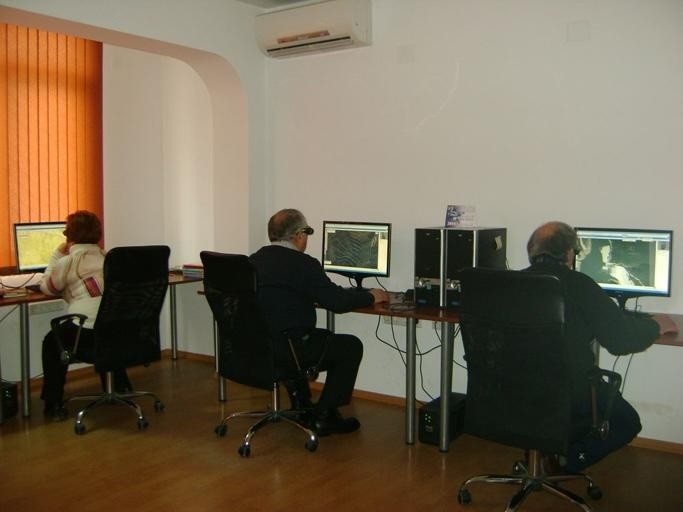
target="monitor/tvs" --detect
[322,221,391,291]
[573,227,673,317]
[14,222,67,272]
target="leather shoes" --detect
[42,398,70,424]
[287,397,361,437]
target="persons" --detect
[520,222,677,473]
[248,209,390,436]
[40,211,132,421]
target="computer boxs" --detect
[413,227,486,308]
[445,228,507,306]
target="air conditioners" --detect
[256,0,373,58]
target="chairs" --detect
[197,251,335,458]
[456,267,622,512]
[51,246,171,435]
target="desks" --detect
[0,266,220,418]
[417,289,683,453]
[195,286,417,445]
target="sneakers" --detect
[543,455,562,486]
